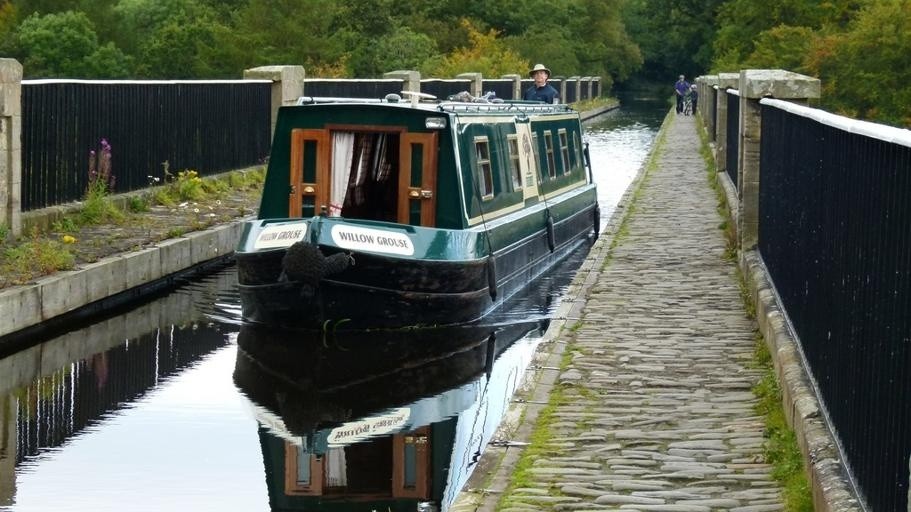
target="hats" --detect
[529,64,552,80]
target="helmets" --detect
[691,84,698,89]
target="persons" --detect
[689,85,698,114]
[521,63,561,104]
[674,74,692,114]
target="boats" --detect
[232,80,603,325]
[227,315,552,511]
